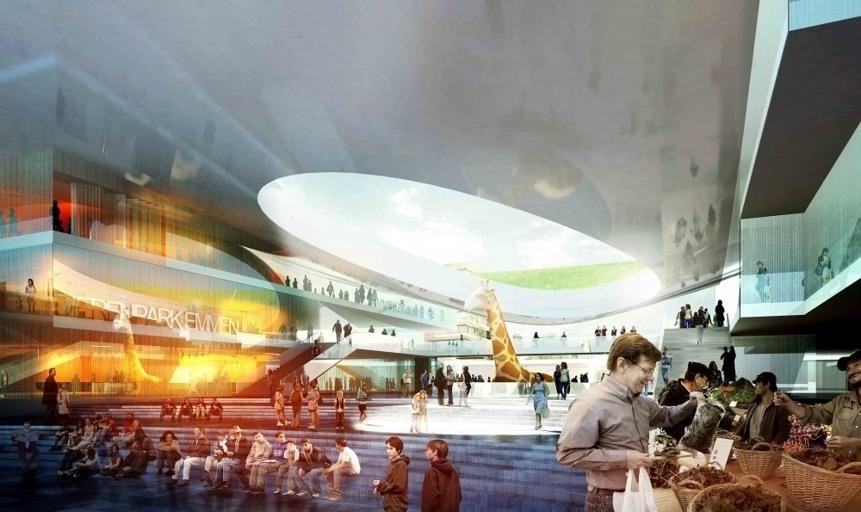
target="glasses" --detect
[629,359,656,376]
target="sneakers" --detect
[166,479,189,486]
[203,479,265,495]
[273,490,343,501]
[277,421,291,426]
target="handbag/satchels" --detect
[566,385,570,393]
[543,407,550,418]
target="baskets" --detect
[668,429,861,512]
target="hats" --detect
[688,363,713,383]
[753,372,776,384]
[837,351,861,371]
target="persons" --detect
[1,199,858,510]
